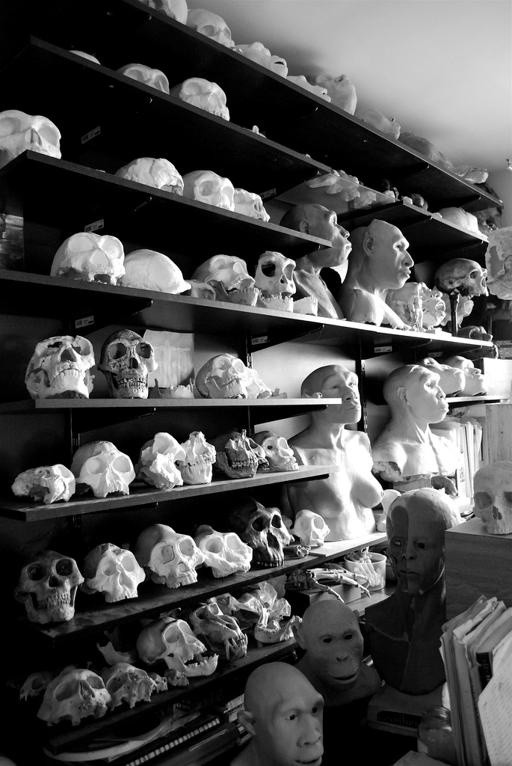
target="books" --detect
[437,594,512,765]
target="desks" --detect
[446,506,511,620]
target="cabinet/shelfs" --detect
[1,0,510,766]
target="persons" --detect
[444,356,487,397]
[364,486,468,695]
[291,599,383,709]
[276,200,352,321]
[334,217,414,330]
[285,365,385,545]
[226,662,326,766]
[371,361,468,481]
